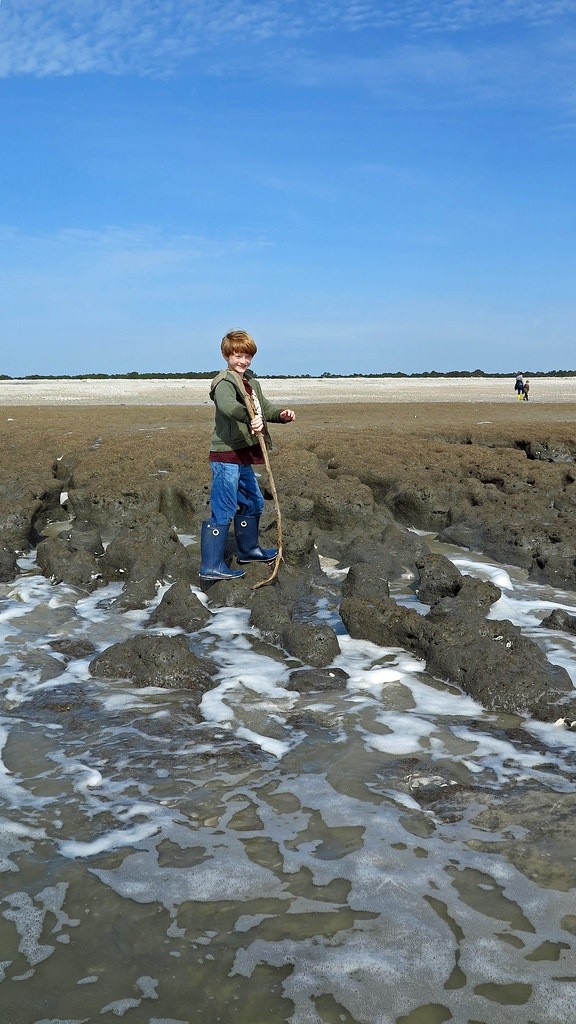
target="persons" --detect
[202,328,296,582]
[515,372,524,402]
[523,380,530,400]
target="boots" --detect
[235,511,279,563]
[199,520,246,582]
